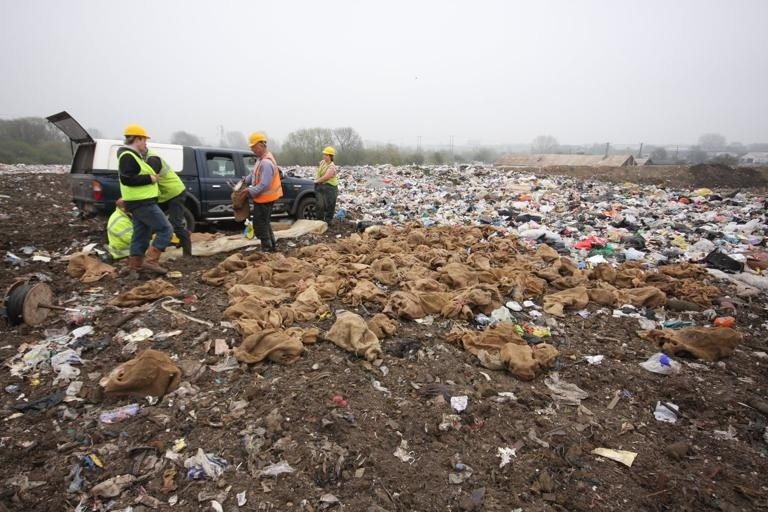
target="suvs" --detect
[42,110,322,231]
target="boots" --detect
[181,240,193,262]
[256,233,276,253]
[141,245,168,274]
[129,256,150,273]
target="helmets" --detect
[322,147,336,156]
[248,132,267,147]
[123,124,151,139]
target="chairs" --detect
[206,158,225,178]
[225,159,235,178]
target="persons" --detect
[115,124,175,274]
[313,146,339,225]
[238,133,284,253]
[106,197,133,252]
[139,146,192,262]
